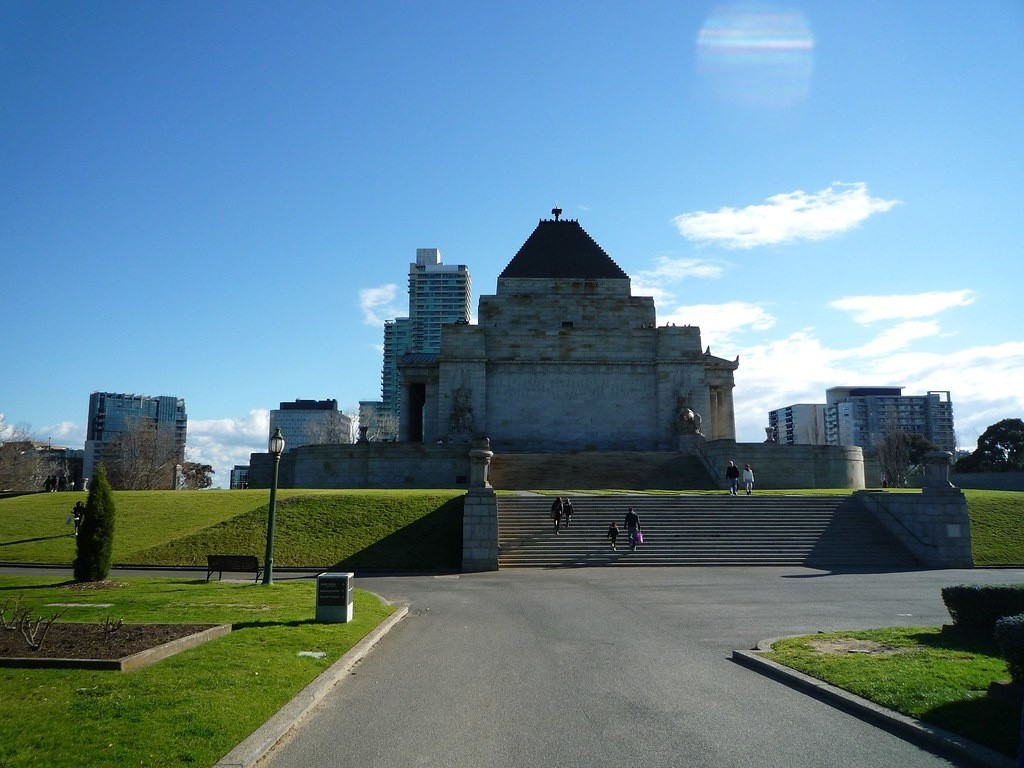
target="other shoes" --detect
[733,491,736,495]
[747,491,751,495]
[566,524,569,527]
[556,532,559,535]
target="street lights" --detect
[260,423,286,586]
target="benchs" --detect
[204,554,263,583]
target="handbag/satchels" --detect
[637,531,643,543]
[551,512,554,519]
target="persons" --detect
[455,319,468,324]
[43,474,75,492]
[551,497,563,534]
[726,461,740,495]
[608,521,618,551]
[70,501,85,535]
[624,508,641,551]
[564,498,573,527]
[743,465,754,495]
